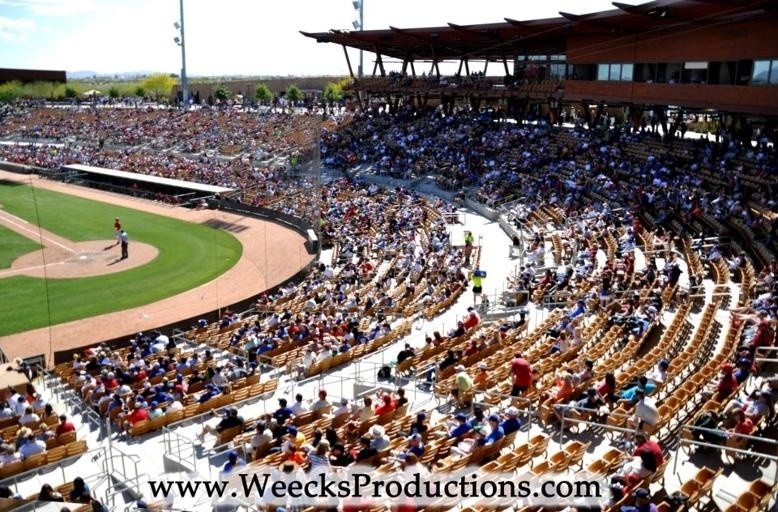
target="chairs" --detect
[0,238,352,511]
[352,111,778,512]
[0,102,352,226]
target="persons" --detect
[0,291,778,508]
[264,217,778,294]
[120,230,129,260]
[0,66,778,218]
[113,217,122,245]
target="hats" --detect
[228,451,238,459]
[235,358,256,367]
[408,433,422,440]
[658,359,668,367]
[454,413,466,420]
[205,384,213,388]
[369,424,385,438]
[487,406,518,421]
[454,362,487,371]
[137,363,182,406]
[16,425,32,438]
[417,412,425,419]
[721,350,751,370]
[287,427,297,433]
